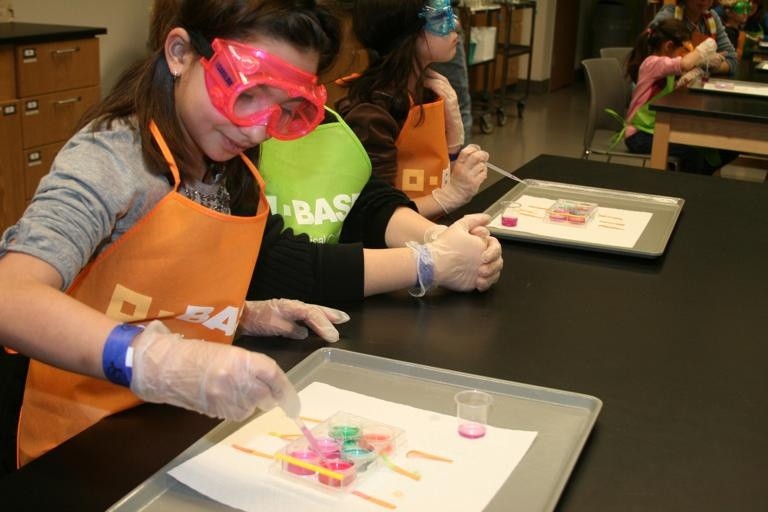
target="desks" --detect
[12,151,768,511]
[647,82,768,174]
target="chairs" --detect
[579,46,681,174]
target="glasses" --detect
[732,3,752,15]
[421,0,458,36]
[677,41,693,56]
[193,38,330,140]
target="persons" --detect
[433,0,473,143]
[0,0,350,512]
[623,0,737,110]
[621,16,740,175]
[334,0,492,216]
[711,0,768,64]
[240,2,507,317]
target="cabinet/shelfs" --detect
[456,0,537,117]
[0,38,102,237]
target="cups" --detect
[500,201,523,227]
[702,72,710,82]
[454,390,494,438]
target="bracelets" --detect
[415,243,434,291]
[101,318,147,384]
